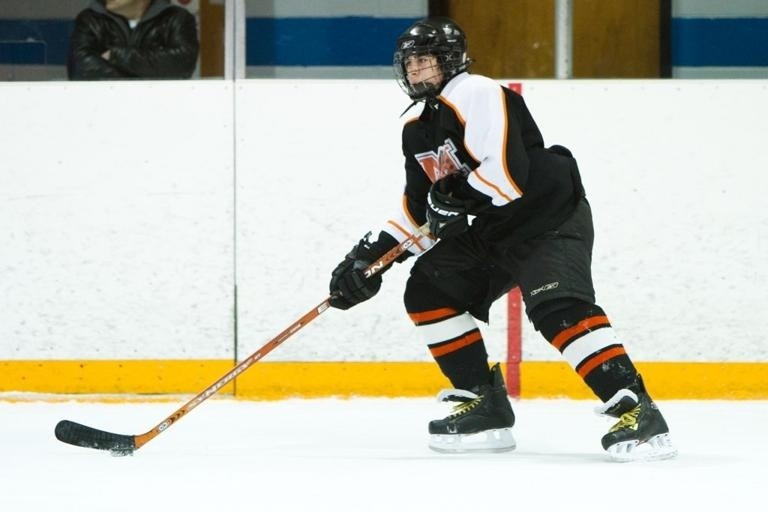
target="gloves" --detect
[330,231,393,309]
[426,172,491,239]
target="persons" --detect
[329,16,677,463]
[67,2,199,81]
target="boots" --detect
[594,373,668,450]
[429,363,514,433]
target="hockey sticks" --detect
[56,224,433,457]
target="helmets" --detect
[394,17,476,99]
